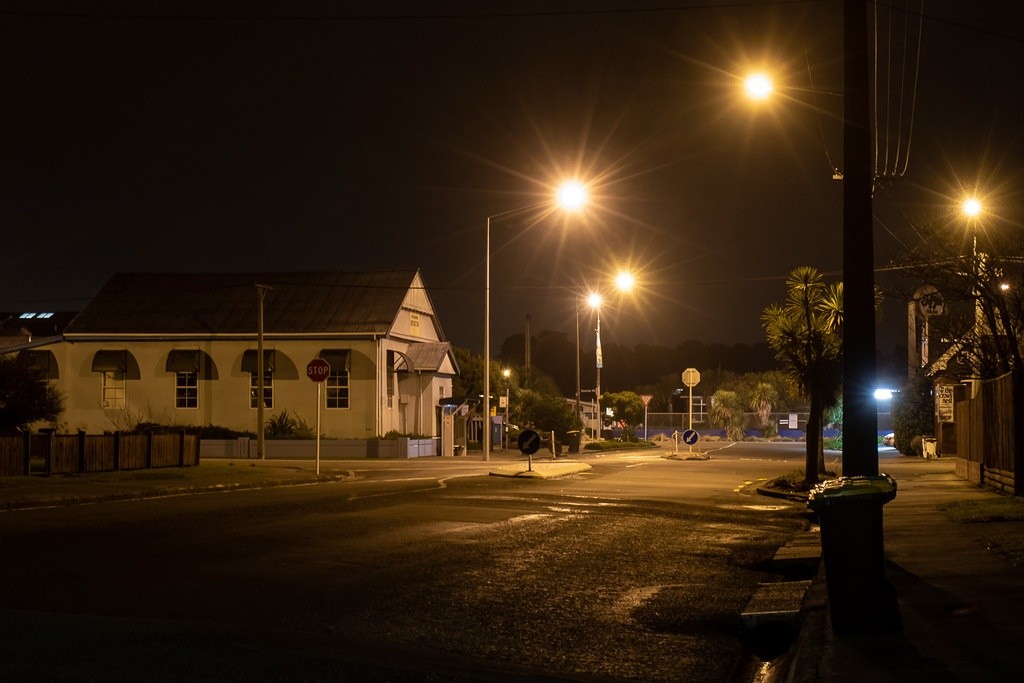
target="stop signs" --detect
[307,358,331,382]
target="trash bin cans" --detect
[805,471,896,637]
[567,431,582,452]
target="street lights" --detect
[503,369,512,450]
[482,184,589,462]
[588,293,603,439]
